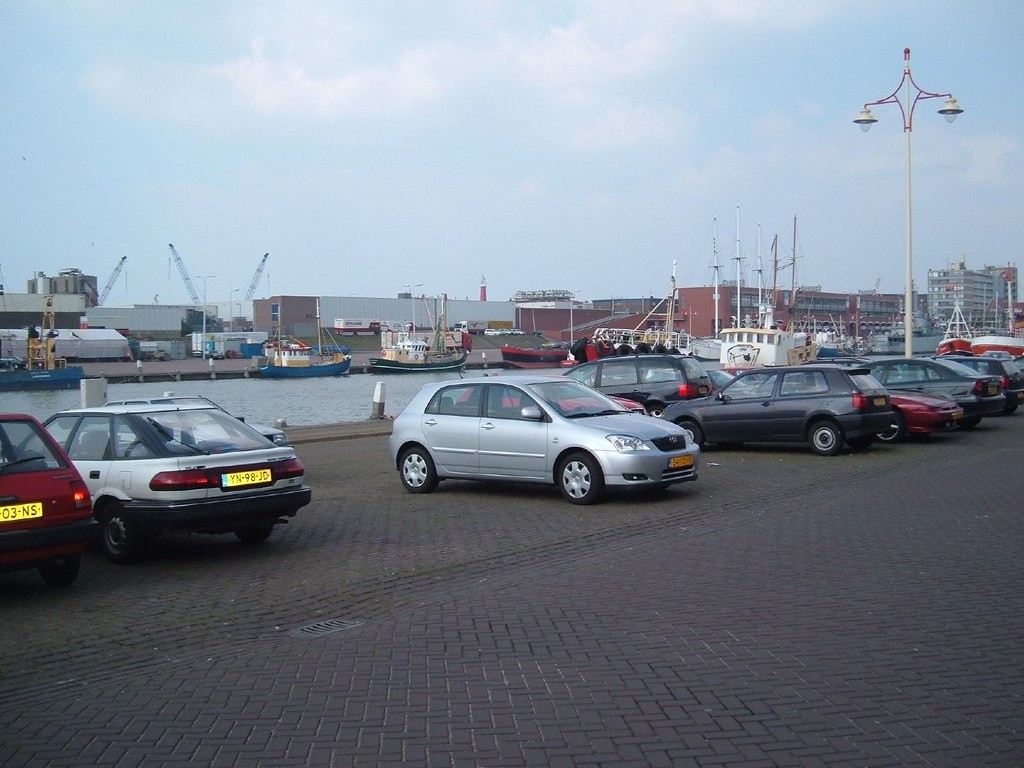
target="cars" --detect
[390,375,701,504]
[704,369,754,394]
[854,358,1024,430]
[876,392,970,444]
[0,393,313,588]
[888,328,905,343]
[457,376,649,420]
[208,350,225,360]
[721,366,768,382]
[0,357,28,369]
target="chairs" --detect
[80,431,109,455]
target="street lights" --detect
[402,283,423,334]
[569,289,582,348]
[852,46,964,355]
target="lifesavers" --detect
[668,348,681,354]
[652,344,668,353]
[595,341,615,357]
[570,337,587,355]
[414,354,418,360]
[614,344,635,356]
[381,351,386,357]
[635,342,652,354]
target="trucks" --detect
[454,321,488,336]
[334,318,416,337]
[380,331,472,353]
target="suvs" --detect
[660,364,897,457]
[562,353,716,419]
[752,357,902,395]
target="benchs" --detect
[430,388,480,415]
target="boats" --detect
[936,302,973,358]
[969,270,1024,358]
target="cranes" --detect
[99,242,279,311]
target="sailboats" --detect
[257,298,355,381]
[369,295,470,375]
[484,206,876,368]
